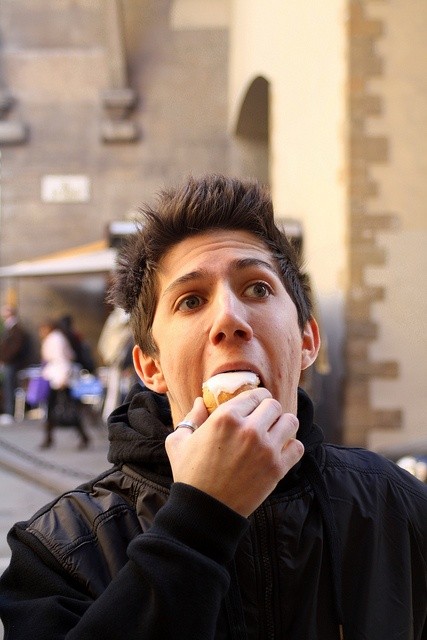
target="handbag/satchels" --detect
[26,377,50,405]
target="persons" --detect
[0,176,427,640]
[39,314,93,450]
[0,304,26,424]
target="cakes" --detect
[199,370,261,415]
